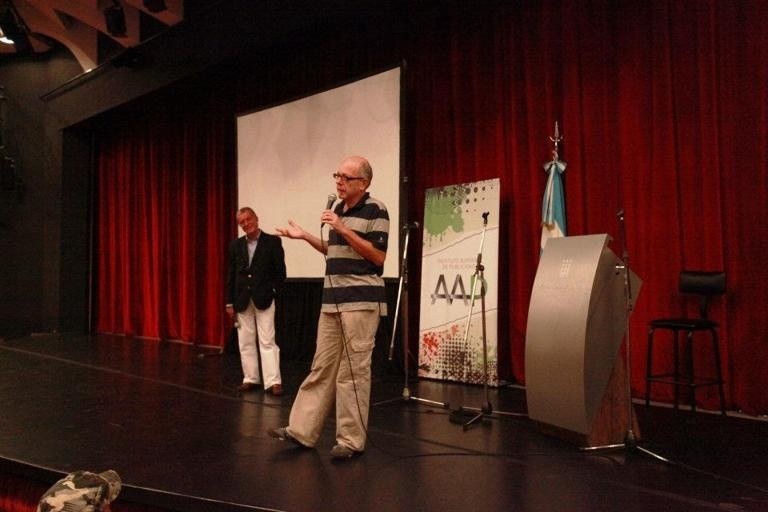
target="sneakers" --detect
[330,444,353,459]
[268,426,307,449]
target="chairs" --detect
[644,271,728,418]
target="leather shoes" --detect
[238,384,251,390]
[272,384,282,395]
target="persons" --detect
[223,206,285,395]
[265,156,390,459]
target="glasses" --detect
[334,172,364,182]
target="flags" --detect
[538,163,568,257]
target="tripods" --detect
[460,211,529,429]
[576,210,672,466]
[370,229,451,408]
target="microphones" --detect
[320,194,337,227]
[231,317,240,328]
[401,221,420,228]
